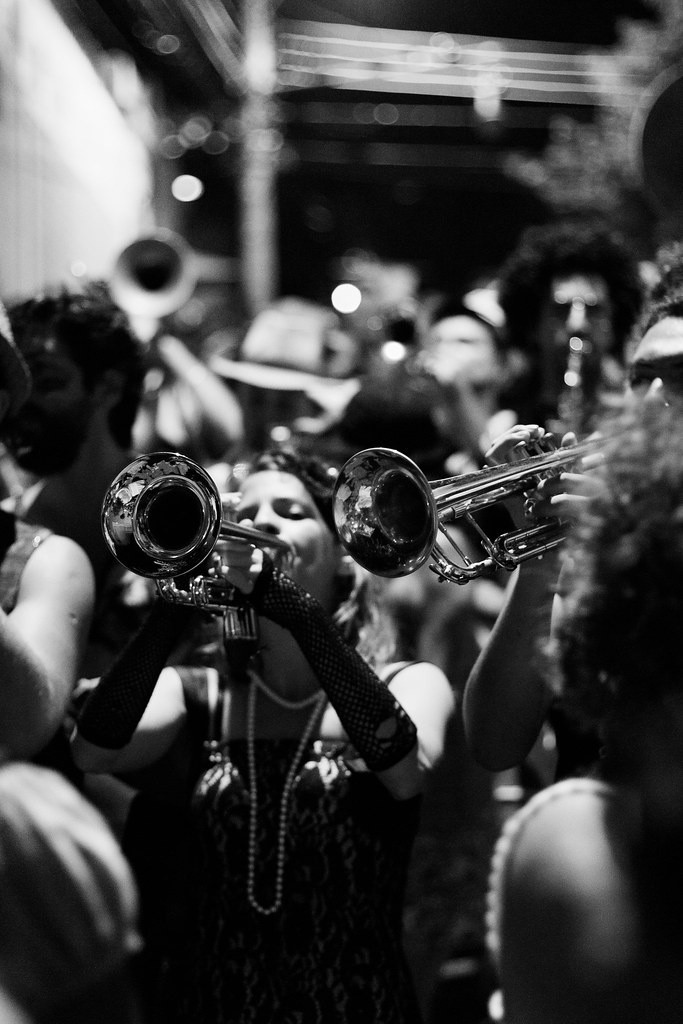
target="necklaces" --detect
[246,670,326,917]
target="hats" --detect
[0,297,31,404]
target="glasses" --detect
[628,351,683,396]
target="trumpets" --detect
[99,452,298,611]
[332,424,648,585]
[112,225,202,314]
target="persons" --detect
[0,56,683,1024]
